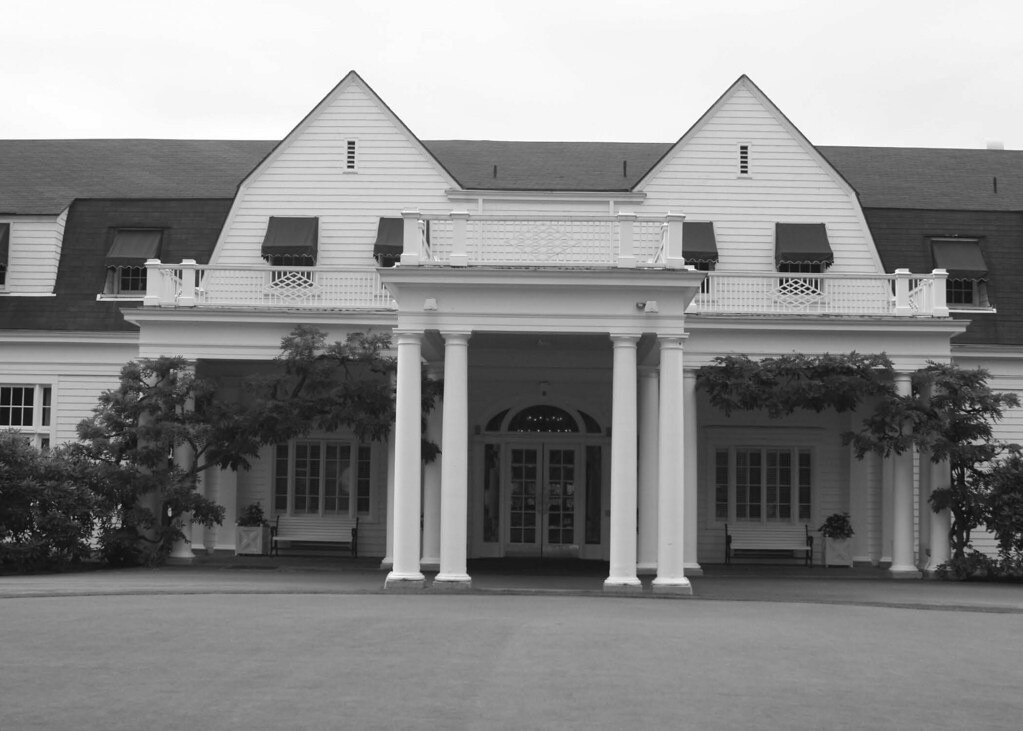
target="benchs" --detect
[721,521,815,569]
[270,515,361,558]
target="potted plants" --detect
[234,503,272,556]
[817,515,854,569]
